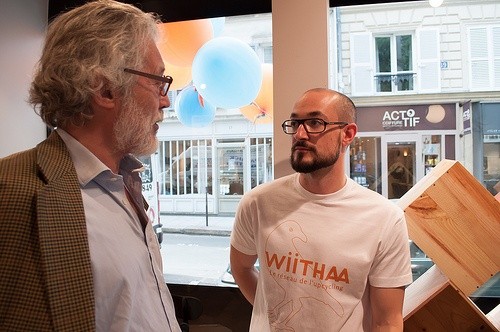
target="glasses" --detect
[282,118,348,134]
[124,68,173,96]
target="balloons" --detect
[156,17,273,128]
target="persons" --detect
[230,88,413,332]
[0,0,182,332]
